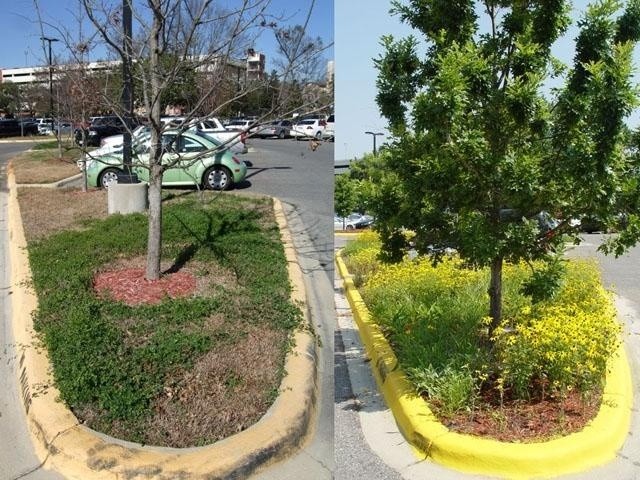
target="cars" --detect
[582,213,629,234]
[74,116,259,192]
[0,116,81,137]
[255,113,334,141]
[335,212,379,232]
[503,210,564,245]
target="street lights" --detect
[366,131,384,155]
[40,36,60,131]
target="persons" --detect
[319,119,326,126]
